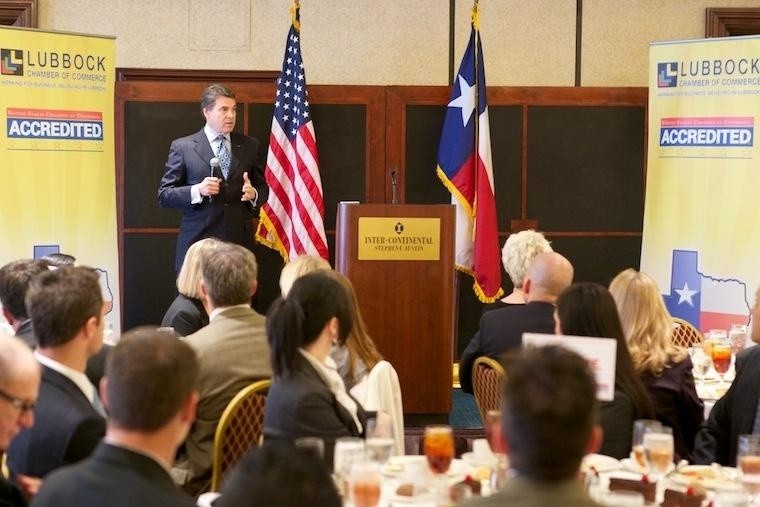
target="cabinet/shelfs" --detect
[114,79,649,363]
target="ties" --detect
[216,133,232,180]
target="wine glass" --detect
[686,321,748,386]
[292,417,760,507]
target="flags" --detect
[255,3,330,265]
[436,14,504,304]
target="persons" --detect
[158,85,269,271]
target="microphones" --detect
[208,157,220,203]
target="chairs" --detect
[209,379,272,491]
[672,317,703,348]
[472,356,507,426]
